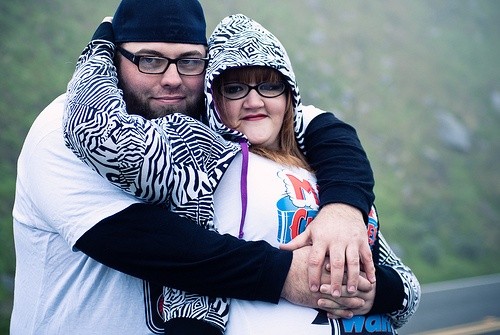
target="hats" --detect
[111,0,208,46]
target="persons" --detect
[9,0,376,335]
[63,14,421,335]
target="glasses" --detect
[115,45,209,76]
[220,80,288,100]
